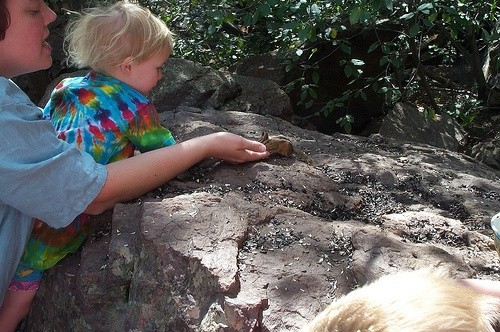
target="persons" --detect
[1,2,177,331]
[1,1,269,332]
